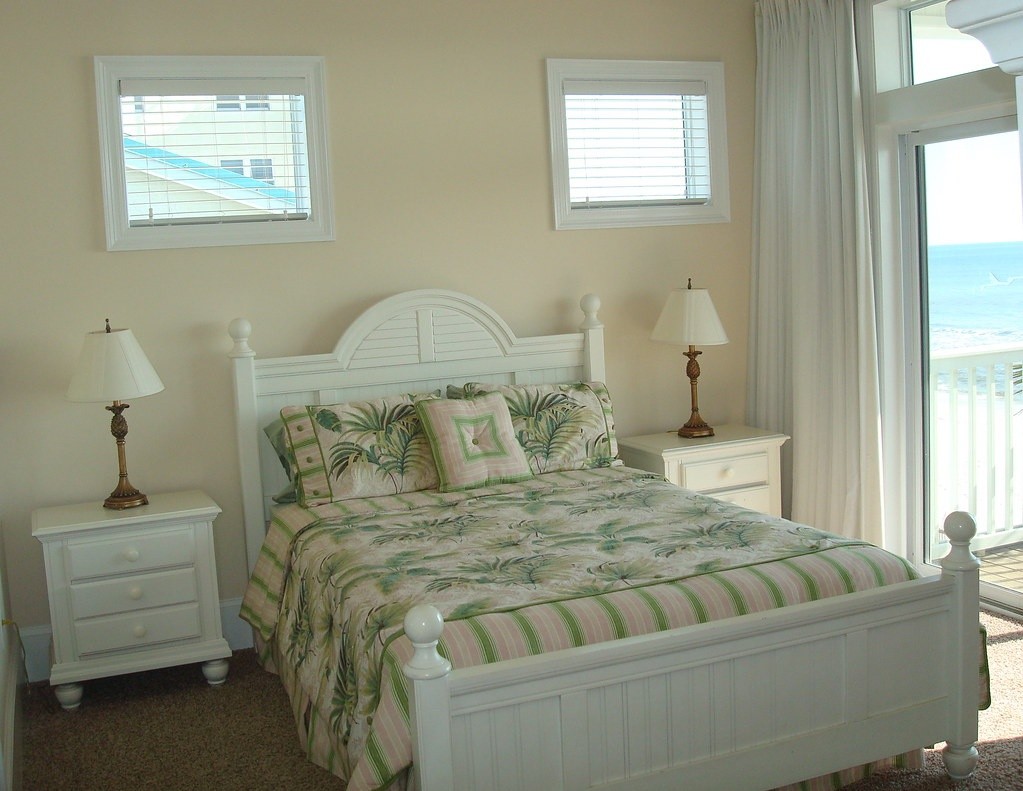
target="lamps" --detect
[648,277,732,438]
[63,316,166,510]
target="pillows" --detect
[261,381,624,509]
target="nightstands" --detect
[31,491,235,709]
[616,423,792,518]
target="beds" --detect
[226,286,992,791]
